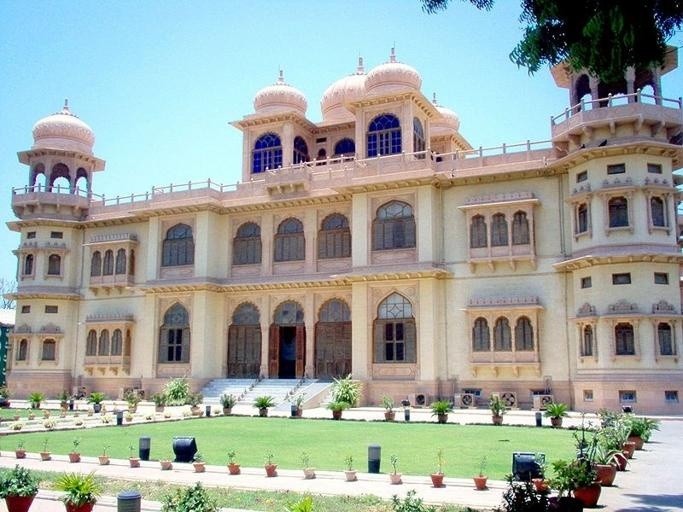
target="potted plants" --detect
[0,433,218,512]
[0,377,203,430]
[218,373,452,428]
[226,448,447,512]
[473,394,660,512]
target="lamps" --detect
[206,406,211,416]
